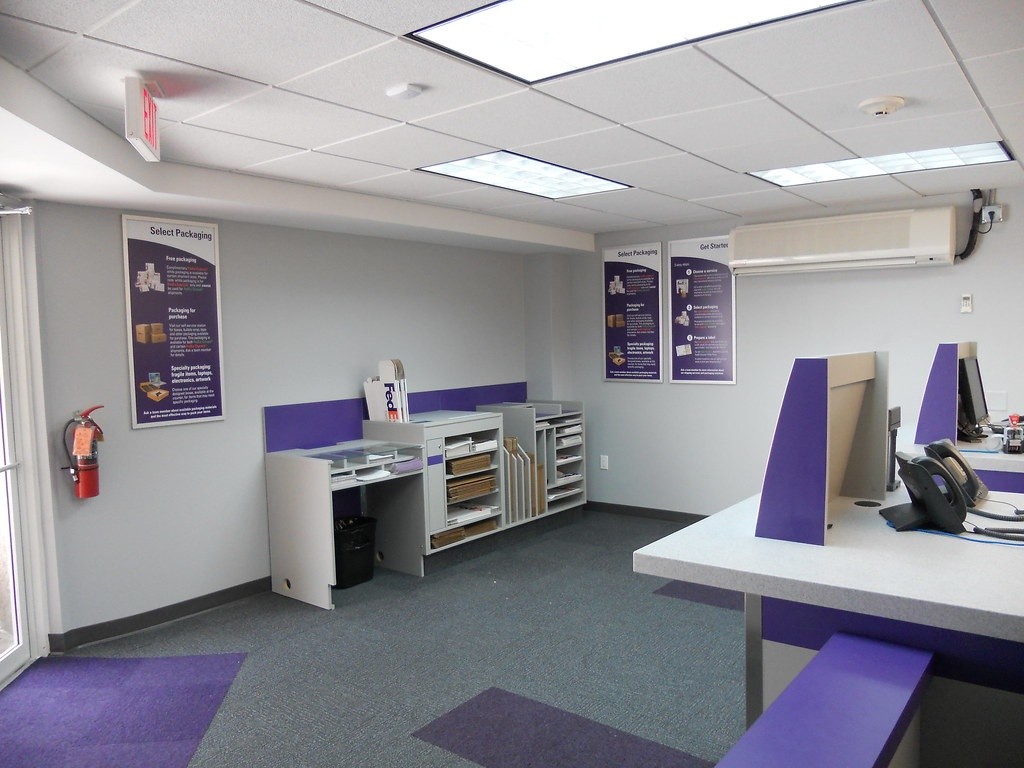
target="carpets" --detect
[410,687,716,768]
[0,653,248,768]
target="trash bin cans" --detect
[334,516,376,589]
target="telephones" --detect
[924,437,989,507]
[878,452,967,534]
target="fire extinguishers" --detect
[62,405,103,497]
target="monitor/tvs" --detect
[958,356,989,443]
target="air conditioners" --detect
[728,207,956,277]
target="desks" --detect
[632,425,1024,731]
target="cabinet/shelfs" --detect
[264,400,587,611]
